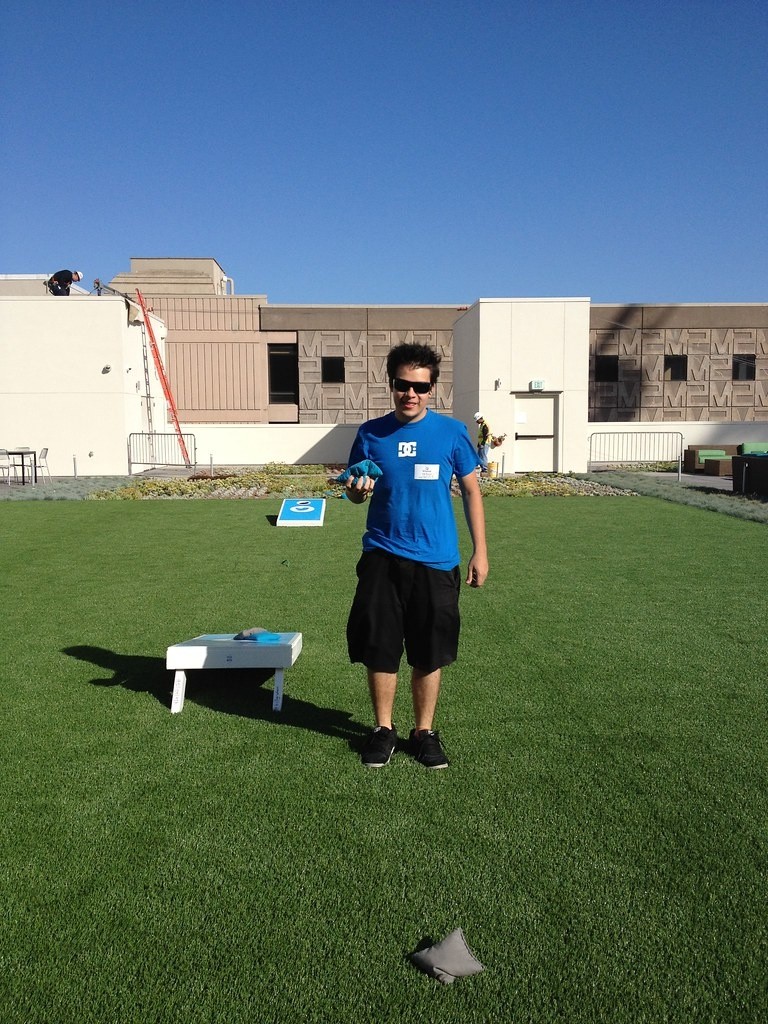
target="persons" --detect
[473,412,492,473]
[48,270,83,296]
[344,344,489,768]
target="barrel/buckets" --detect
[487,461,499,478]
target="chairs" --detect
[0,448,19,486]
[26,448,52,485]
[7,447,32,483]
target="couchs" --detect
[684,442,768,476]
[731,452,768,499]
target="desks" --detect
[0,451,38,486]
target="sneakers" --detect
[363,722,398,767]
[409,728,449,769]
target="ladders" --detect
[136,287,192,469]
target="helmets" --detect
[474,412,483,422]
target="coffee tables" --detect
[705,458,732,476]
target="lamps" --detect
[105,365,111,372]
[497,378,501,387]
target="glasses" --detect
[393,377,432,394]
